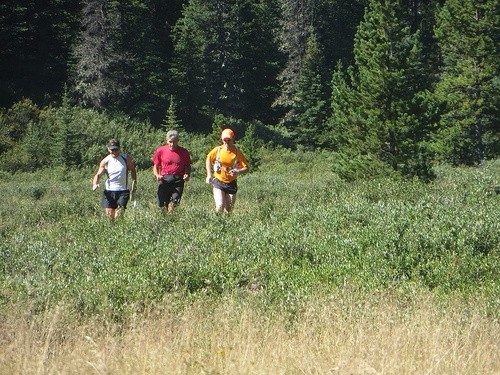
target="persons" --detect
[204,128,248,216]
[91,139,137,220]
[150,130,192,216]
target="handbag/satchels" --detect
[213,160,222,173]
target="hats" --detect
[166,130,179,141]
[221,129,235,140]
[108,139,120,150]
